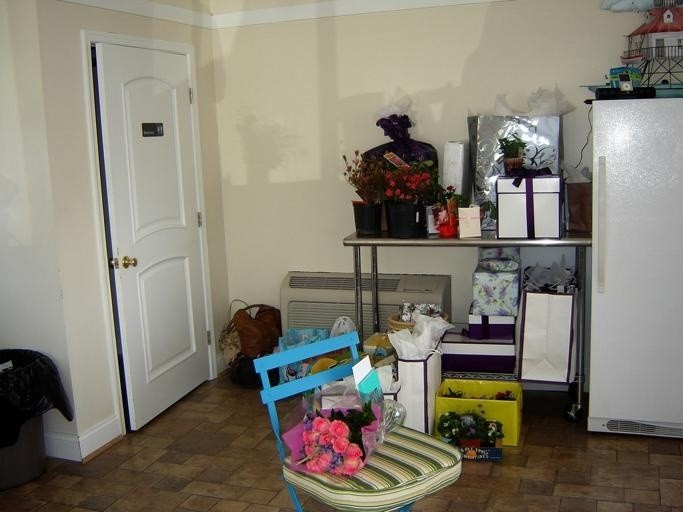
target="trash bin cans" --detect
[0,350,46,492]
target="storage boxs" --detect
[471,265,520,316]
[467,303,515,341]
[495,176,563,238]
[442,324,517,374]
[435,378,523,448]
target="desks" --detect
[342,231,592,420]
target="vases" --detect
[385,203,424,239]
[458,438,481,449]
[353,202,382,240]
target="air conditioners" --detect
[279,270,449,345]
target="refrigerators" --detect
[584,95,683,443]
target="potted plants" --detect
[498,132,527,177]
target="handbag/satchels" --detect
[218,305,282,365]
[514,266,578,385]
[396,341,444,435]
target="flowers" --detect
[438,412,502,445]
[342,150,383,202]
[277,384,407,479]
[383,161,440,205]
[434,185,456,205]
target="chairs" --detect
[252,330,464,512]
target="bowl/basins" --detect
[388,312,449,334]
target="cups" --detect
[503,155,523,177]
